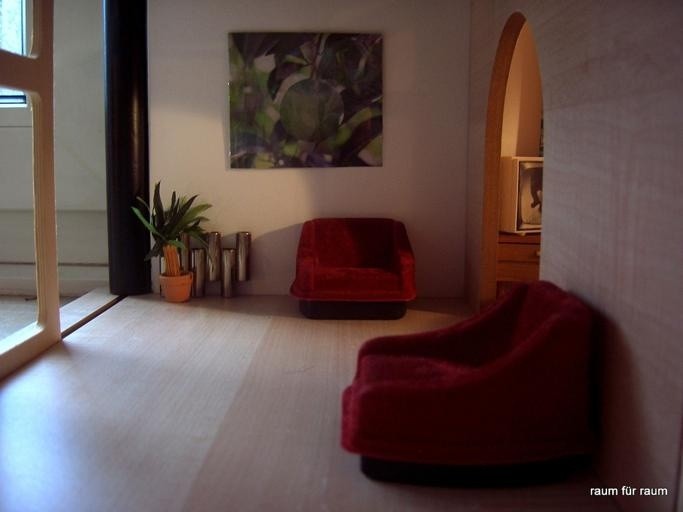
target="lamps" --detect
[340,280,598,491]
[290,216,417,321]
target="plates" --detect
[495,235,541,300]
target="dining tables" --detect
[134,179,214,303]
